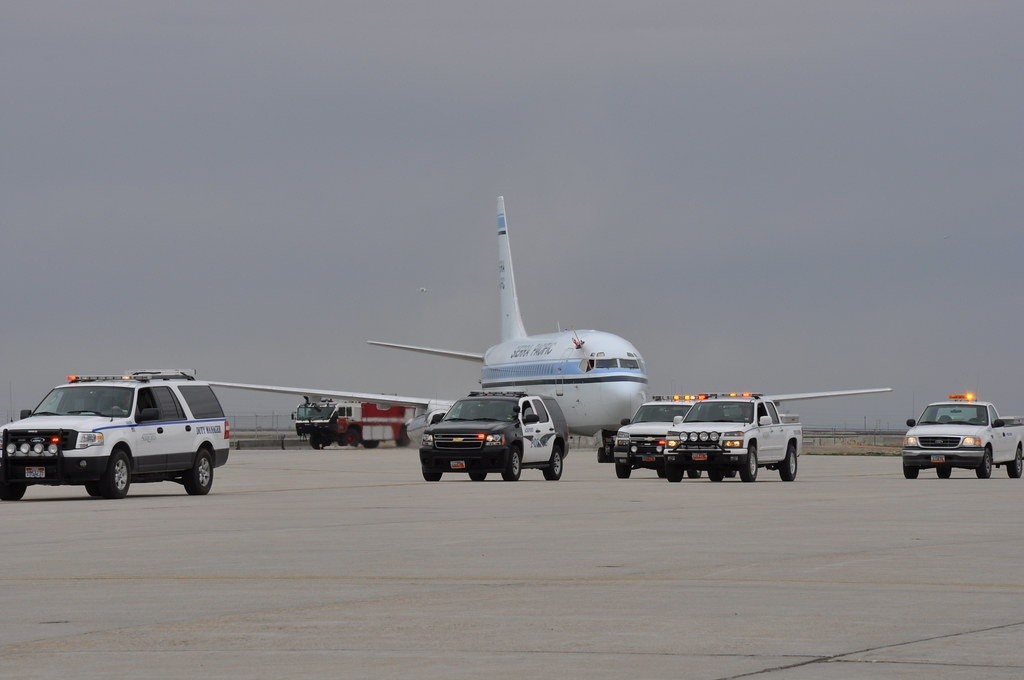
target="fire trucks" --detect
[291,398,420,449]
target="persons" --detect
[969,407,986,422]
[111,392,130,411]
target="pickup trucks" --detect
[901,395,1024,480]
[663,393,804,482]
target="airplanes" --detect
[205,196,893,463]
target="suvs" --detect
[612,395,702,480]
[419,389,570,481]
[0,371,230,502]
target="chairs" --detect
[938,415,952,422]
[708,406,724,418]
[138,394,153,414]
